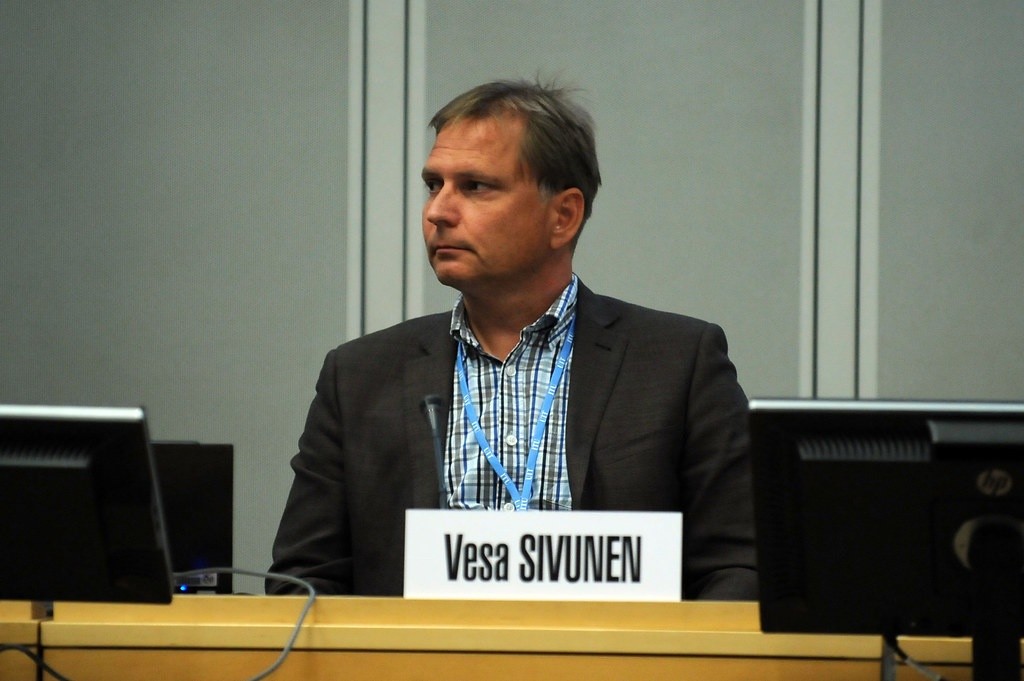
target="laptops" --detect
[151,440,234,596]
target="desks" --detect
[0,593,1024,681]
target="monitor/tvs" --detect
[0,404,175,607]
[746,396,1024,641]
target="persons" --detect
[265,80,758,600]
